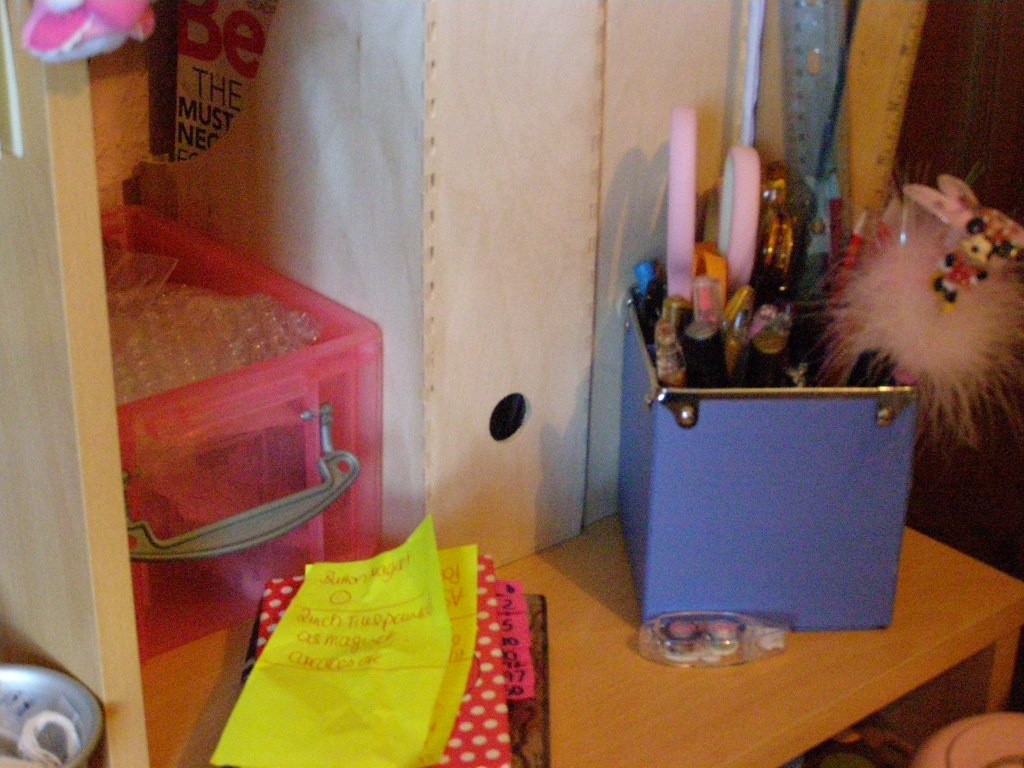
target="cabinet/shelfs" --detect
[134,511,1024,768]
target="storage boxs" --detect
[98,203,387,662]
[619,290,920,631]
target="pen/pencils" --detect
[623,155,1024,388]
